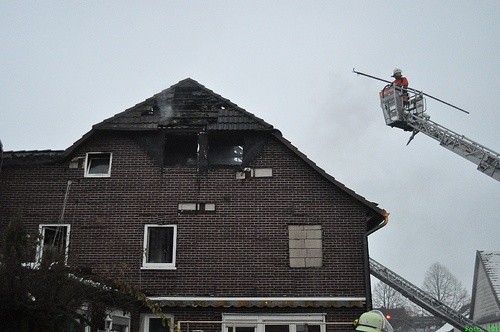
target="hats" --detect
[391,69,402,76]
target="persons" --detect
[390,68,409,108]
